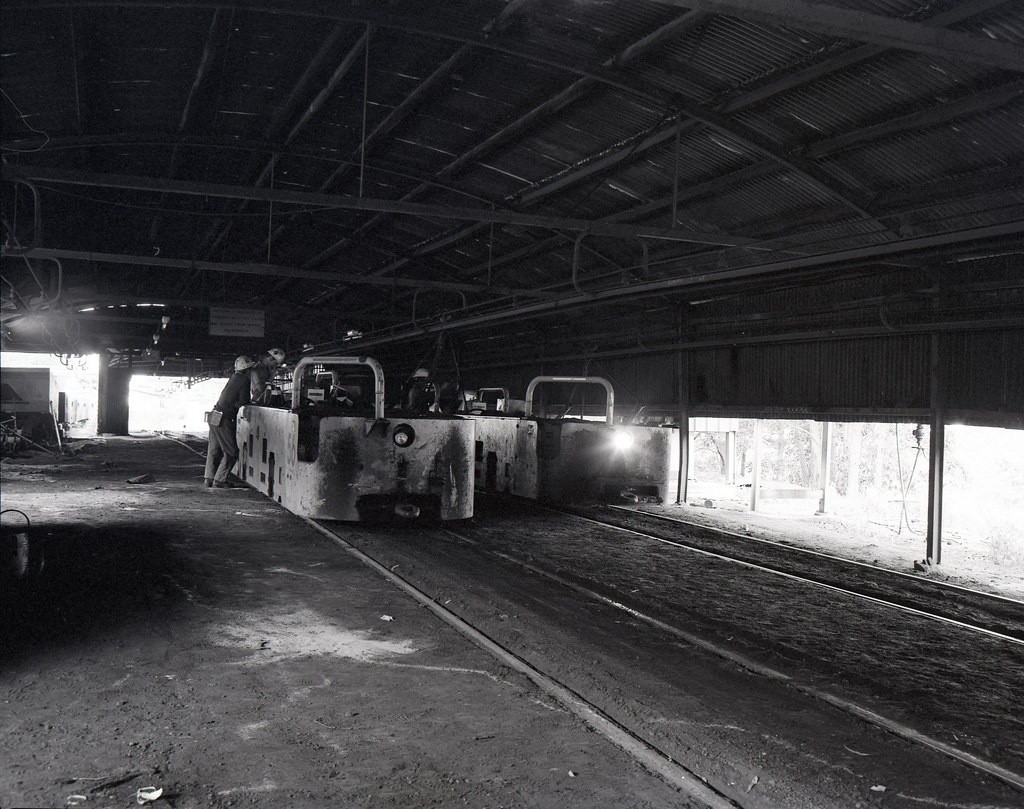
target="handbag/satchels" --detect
[264,385,284,407]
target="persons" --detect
[204,356,255,488]
[408,368,431,412]
[251,349,285,404]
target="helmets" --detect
[233,356,253,370]
[412,368,429,377]
[268,349,285,365]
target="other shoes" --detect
[213,480,234,488]
[205,479,213,487]
